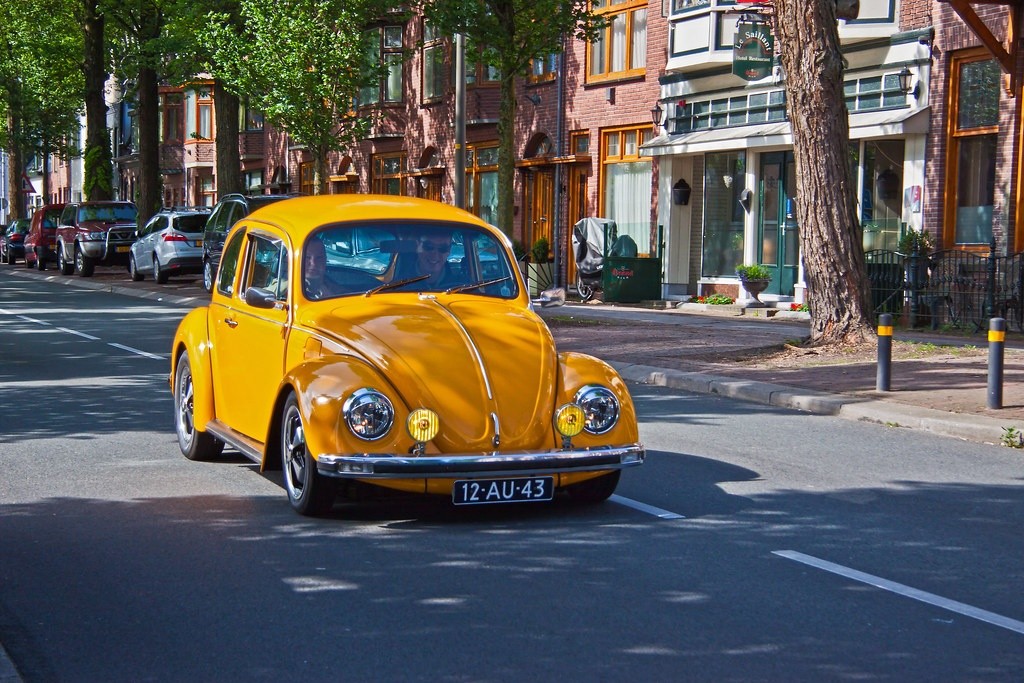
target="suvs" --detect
[129,204,217,284]
[200,193,297,293]
[54,200,143,277]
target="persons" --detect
[263,237,350,301]
[391,226,474,293]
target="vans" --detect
[22,203,69,270]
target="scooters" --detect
[571,217,626,303]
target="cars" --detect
[168,194,645,519]
[0,218,32,266]
[266,220,505,298]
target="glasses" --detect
[419,239,451,254]
